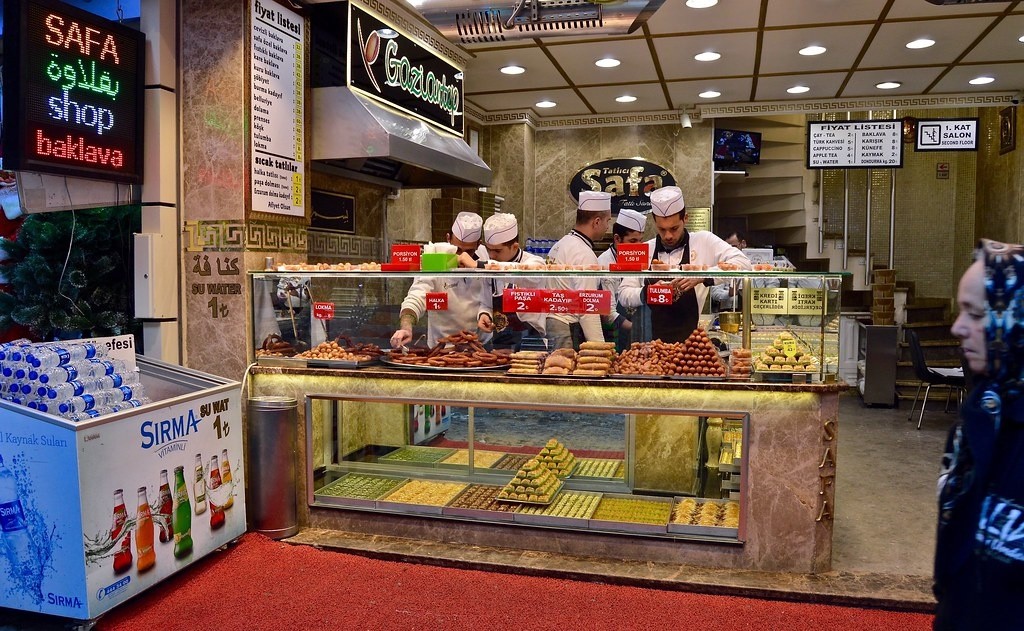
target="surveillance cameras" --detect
[680,113,692,129]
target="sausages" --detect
[387,330,515,368]
[333,335,383,361]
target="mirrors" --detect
[323,399,635,493]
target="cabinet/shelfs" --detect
[856,322,899,407]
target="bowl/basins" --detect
[719,312,741,332]
[671,264,710,272]
[651,264,671,271]
[754,264,772,271]
[718,264,732,271]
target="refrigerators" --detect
[1,353,246,621]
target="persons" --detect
[931,239,1024,631]
[389,186,780,357]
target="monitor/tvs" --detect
[713,128,761,165]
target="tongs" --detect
[414,333,427,346]
[400,343,409,357]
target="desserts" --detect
[318,439,740,530]
[754,330,818,372]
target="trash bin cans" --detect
[247,396,298,539]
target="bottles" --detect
[221,449,234,509]
[436,405,440,425]
[425,405,430,434]
[112,489,132,573]
[419,405,423,415]
[441,405,446,416]
[135,465,195,571]
[534,240,541,257]
[430,405,434,416]
[540,240,548,259]
[548,240,559,254]
[0,339,148,421]
[526,238,535,255]
[209,455,225,528]
[194,454,207,515]
[705,418,722,468]
[414,404,419,432]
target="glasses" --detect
[732,242,742,248]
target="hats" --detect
[578,190,611,211]
[452,212,483,242]
[650,186,684,217]
[484,213,518,245]
[616,209,648,233]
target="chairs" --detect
[907,327,967,430]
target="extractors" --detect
[311,87,491,188]
